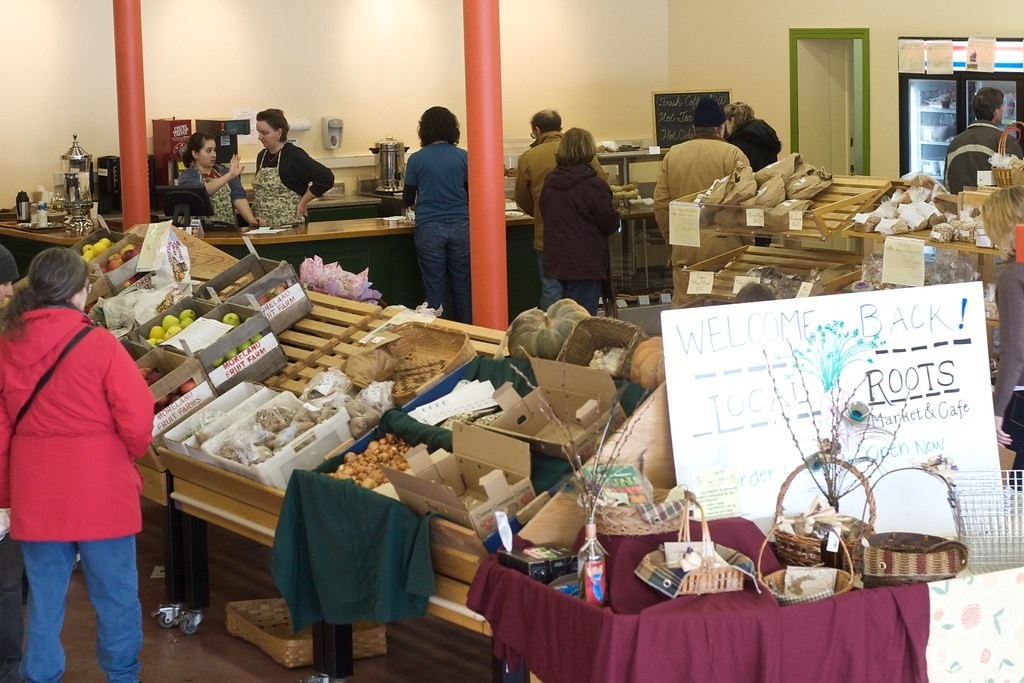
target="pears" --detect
[610,184,639,199]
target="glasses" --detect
[81,283,93,294]
[529,131,535,139]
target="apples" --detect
[147,310,264,368]
[139,367,196,415]
[80,236,150,287]
[259,282,287,306]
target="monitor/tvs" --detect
[155,185,214,216]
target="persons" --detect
[944,87,1023,195]
[654,98,757,309]
[723,101,782,247]
[403,106,472,325]
[0,244,156,683]
[249,108,334,228]
[177,132,266,228]
[982,185,1024,491]
[515,109,620,316]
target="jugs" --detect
[15,190,30,223]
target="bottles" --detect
[37,203,48,228]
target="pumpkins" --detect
[630,337,665,392]
[508,298,590,361]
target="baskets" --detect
[226,598,388,669]
[554,316,649,380]
[845,468,970,586]
[341,321,476,407]
[772,454,877,573]
[991,121,1024,188]
[756,520,854,606]
[656,494,749,597]
[593,483,685,536]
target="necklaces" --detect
[268,147,281,164]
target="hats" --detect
[692,97,727,128]
[973,88,1004,118]
[0,245,20,285]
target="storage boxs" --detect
[88,233,157,296]
[379,420,537,539]
[85,276,113,307]
[184,387,284,464]
[158,302,290,395]
[162,380,267,457]
[126,297,216,352]
[135,347,218,473]
[69,227,130,273]
[117,336,149,360]
[200,391,351,490]
[192,254,314,337]
[469,355,628,465]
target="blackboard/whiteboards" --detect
[652,88,736,152]
[661,279,1008,574]
